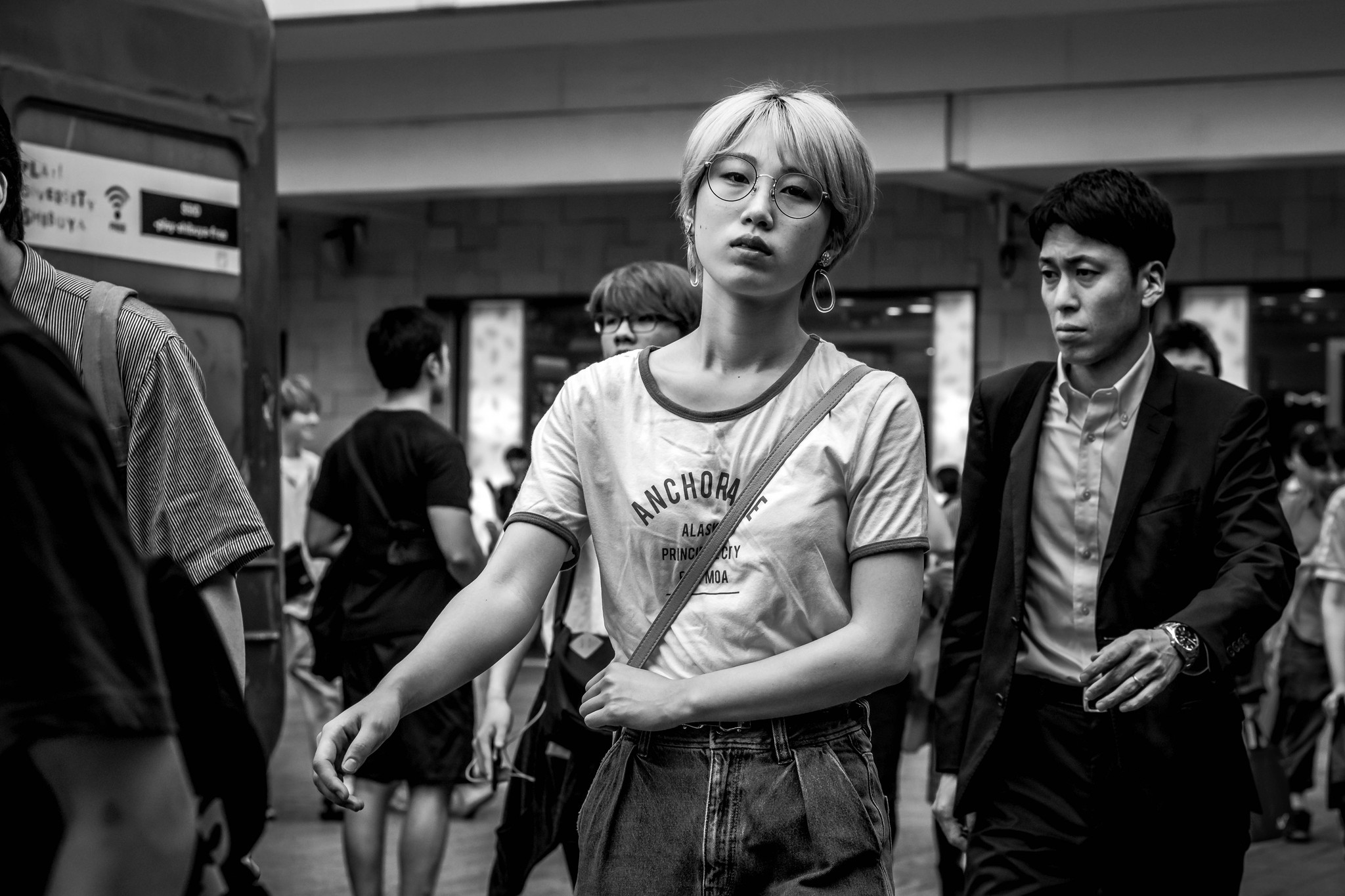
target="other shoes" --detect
[1284,809,1311,841]
[1251,817,1278,840]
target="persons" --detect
[310,83,931,896]
[0,104,277,691]
[466,261,702,896]
[934,169,1302,896]
[0,306,1345,896]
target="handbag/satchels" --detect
[488,625,616,896]
[141,551,270,870]
[343,526,462,637]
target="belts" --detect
[1013,677,1113,715]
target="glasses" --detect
[706,155,833,220]
[593,310,677,333]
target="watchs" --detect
[1154,622,1199,672]
